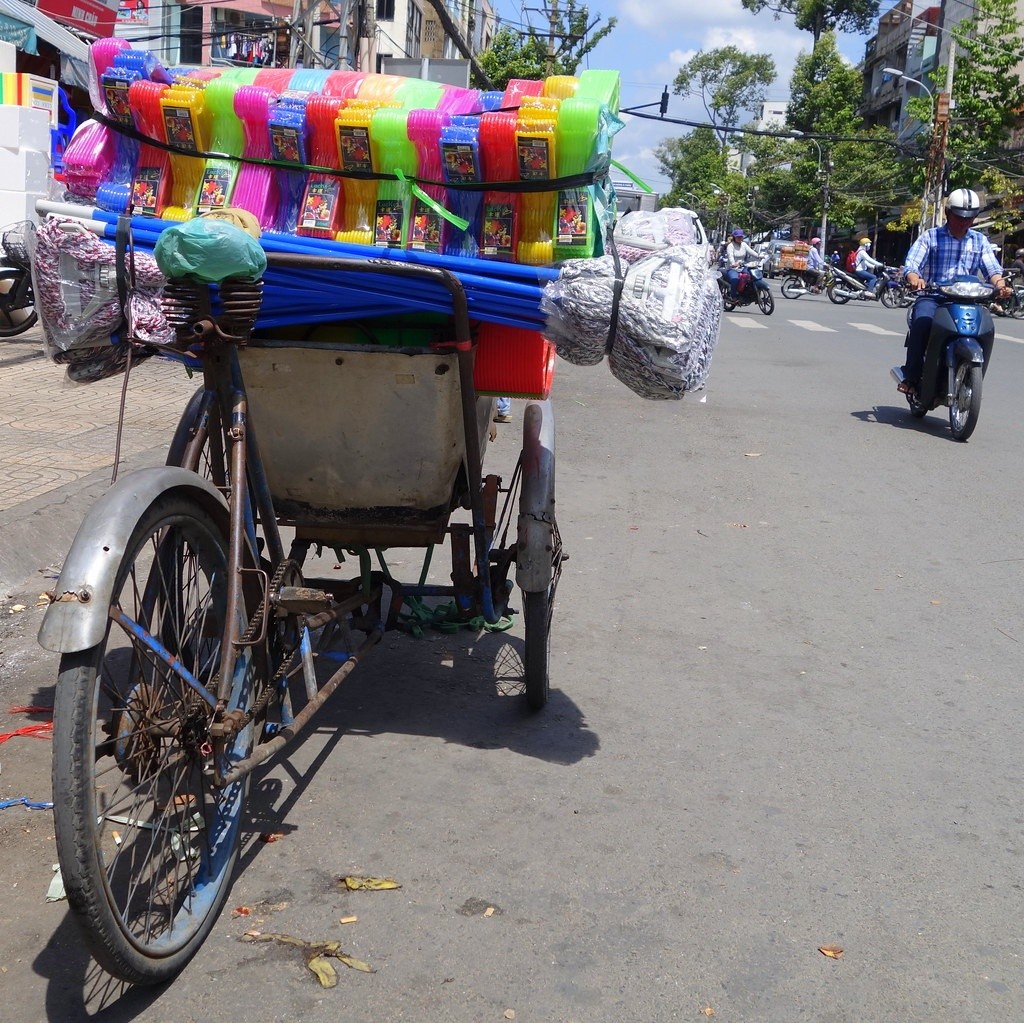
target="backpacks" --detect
[847,249,857,273]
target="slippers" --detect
[899,379,916,394]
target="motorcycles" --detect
[886,268,1019,440]
[717,257,1024,320]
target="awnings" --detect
[0,0,89,66]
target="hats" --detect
[721,241,725,245]
[991,243,1002,252]
[834,251,838,254]
[732,229,743,237]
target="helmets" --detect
[860,238,871,246]
[946,188,981,218]
[1016,248,1024,256]
[810,237,822,244]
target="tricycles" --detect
[29,207,571,985]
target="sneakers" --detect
[863,290,876,298]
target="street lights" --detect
[883,66,934,239]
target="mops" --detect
[29,209,724,401]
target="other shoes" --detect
[730,294,738,301]
[812,284,818,291]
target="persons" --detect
[831,250,840,264]
[897,189,1013,395]
[806,237,829,291]
[854,238,886,298]
[726,230,765,302]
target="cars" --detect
[747,242,772,279]
[763,240,795,279]
